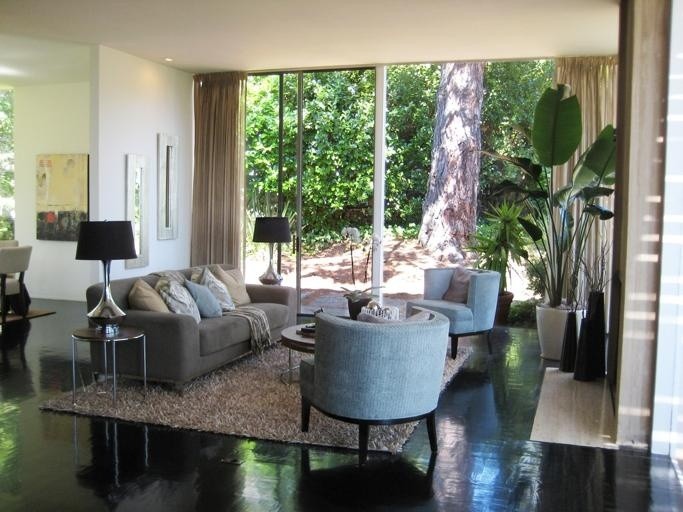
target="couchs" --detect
[84,264,296,390]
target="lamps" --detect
[252,215,292,287]
[76,220,139,334]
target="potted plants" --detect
[468,196,525,325]
[336,283,387,322]
[474,82,617,363]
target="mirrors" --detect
[126,155,152,270]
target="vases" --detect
[586,289,606,381]
[561,311,577,374]
[576,313,598,383]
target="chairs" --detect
[406,263,500,360]
[301,307,451,454]
[0,244,31,317]
[0,239,19,247]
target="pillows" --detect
[182,277,218,321]
[195,267,233,314]
[190,266,203,282]
[125,276,171,331]
[442,265,472,308]
[152,276,200,327]
[210,262,252,305]
[401,310,431,328]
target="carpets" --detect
[0,306,57,324]
[37,315,477,456]
[529,360,621,455]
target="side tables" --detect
[65,328,150,401]
